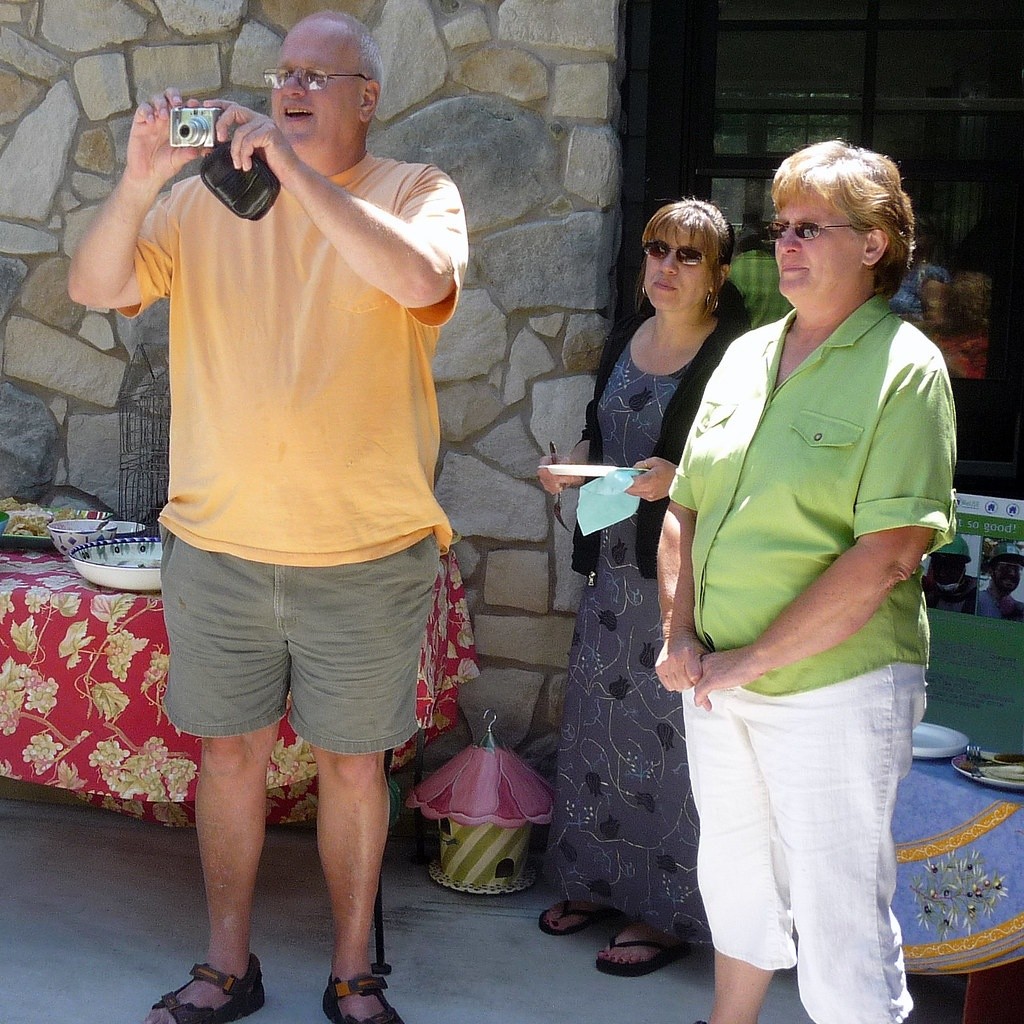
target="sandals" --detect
[144,951,265,1024]
[538,901,622,936]
[596,921,691,977]
[323,973,405,1024]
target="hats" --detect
[986,541,1024,566]
[929,534,971,564]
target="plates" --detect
[912,722,970,759]
[951,755,1024,788]
[538,465,651,476]
[2,508,114,548]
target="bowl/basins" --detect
[112,521,147,538]
[47,520,118,555]
[67,537,162,590]
[0,511,10,537]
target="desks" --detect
[0,546,480,975]
[891,608,1024,1024]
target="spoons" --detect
[550,441,574,533]
[967,744,982,777]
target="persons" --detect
[537,199,754,977]
[68,12,469,1023]
[923,532,1024,623]
[657,139,958,1024]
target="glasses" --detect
[641,239,707,266]
[262,66,373,91]
[768,219,853,240]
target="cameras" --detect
[169,107,223,148]
